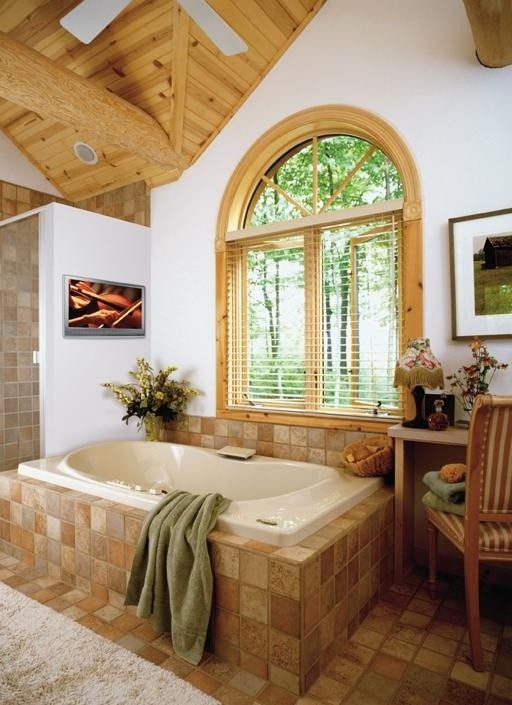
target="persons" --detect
[68,309,121,328]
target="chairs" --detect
[425,392,511,673]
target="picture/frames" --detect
[62,273,146,337]
[446,208,512,340]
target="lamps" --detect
[393,335,443,429]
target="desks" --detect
[387,420,471,585]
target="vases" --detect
[143,415,163,442]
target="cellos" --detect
[69,282,141,330]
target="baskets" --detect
[339,435,395,478]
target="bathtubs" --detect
[16,439,381,547]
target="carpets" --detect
[0,583,221,705]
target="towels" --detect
[119,489,235,667]
[420,463,467,517]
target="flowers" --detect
[99,353,202,435]
[445,333,509,418]
